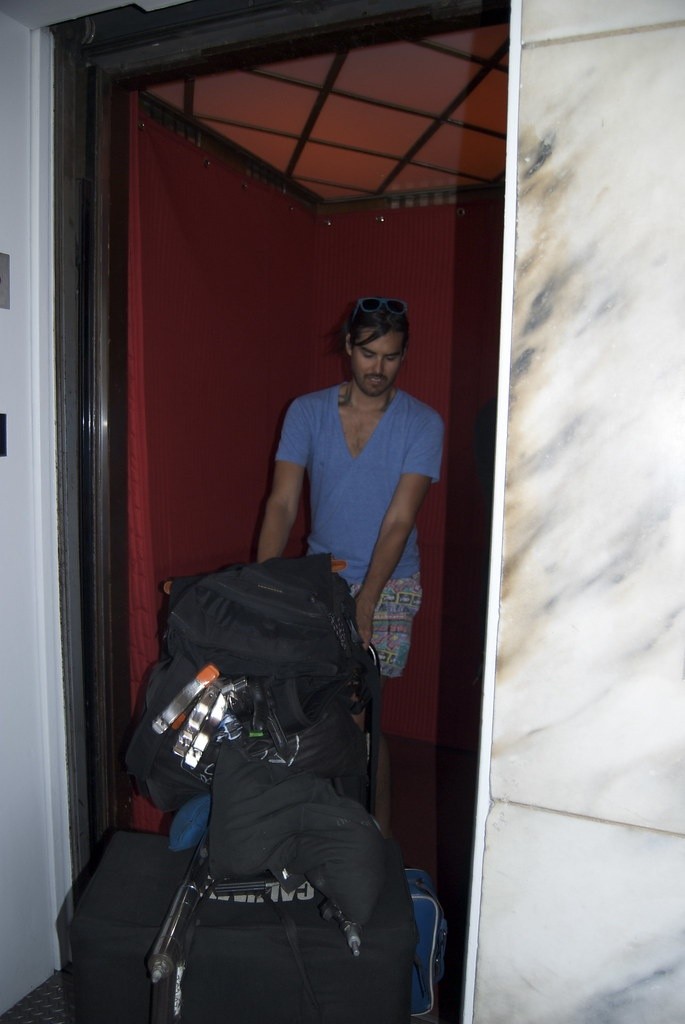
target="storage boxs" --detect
[66,827,420,1024]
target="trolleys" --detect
[354,639,382,812]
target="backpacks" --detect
[120,552,375,814]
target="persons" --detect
[259,298,445,836]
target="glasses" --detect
[353,297,408,320]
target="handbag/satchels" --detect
[68,832,447,1024]
